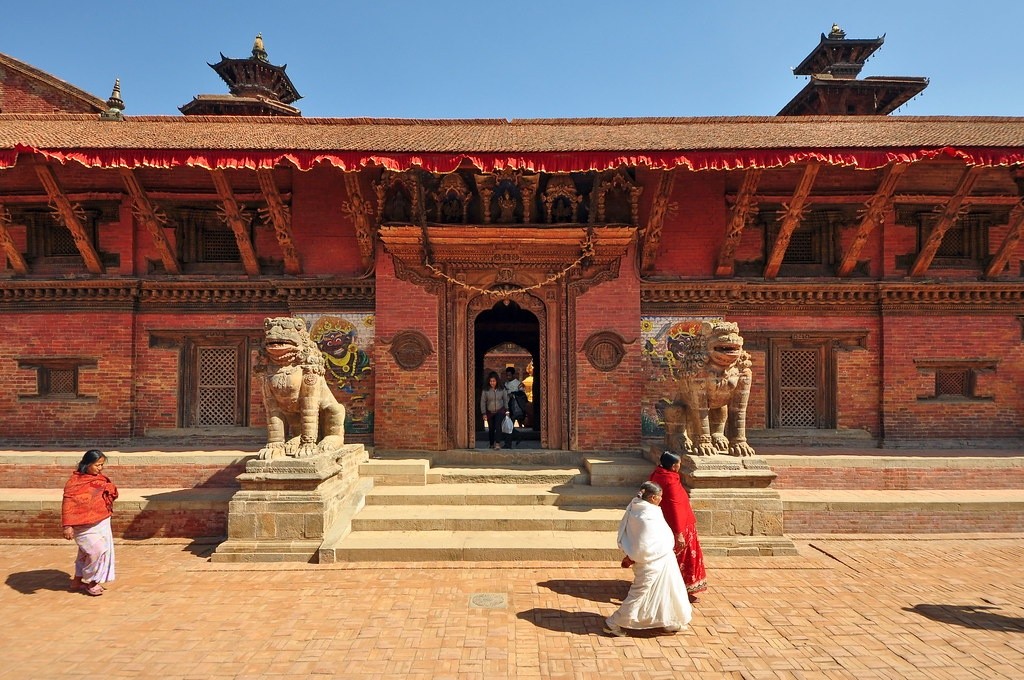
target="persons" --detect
[61,449,119,596]
[502,367,526,448]
[603,481,692,637]
[649,451,707,602]
[480,373,510,449]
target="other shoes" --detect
[516,439,521,448]
[495,443,500,449]
[501,445,511,450]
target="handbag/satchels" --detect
[484,418,489,432]
[501,411,513,434]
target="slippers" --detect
[67,579,85,592]
[84,585,103,596]
[664,623,687,634]
[602,618,629,637]
